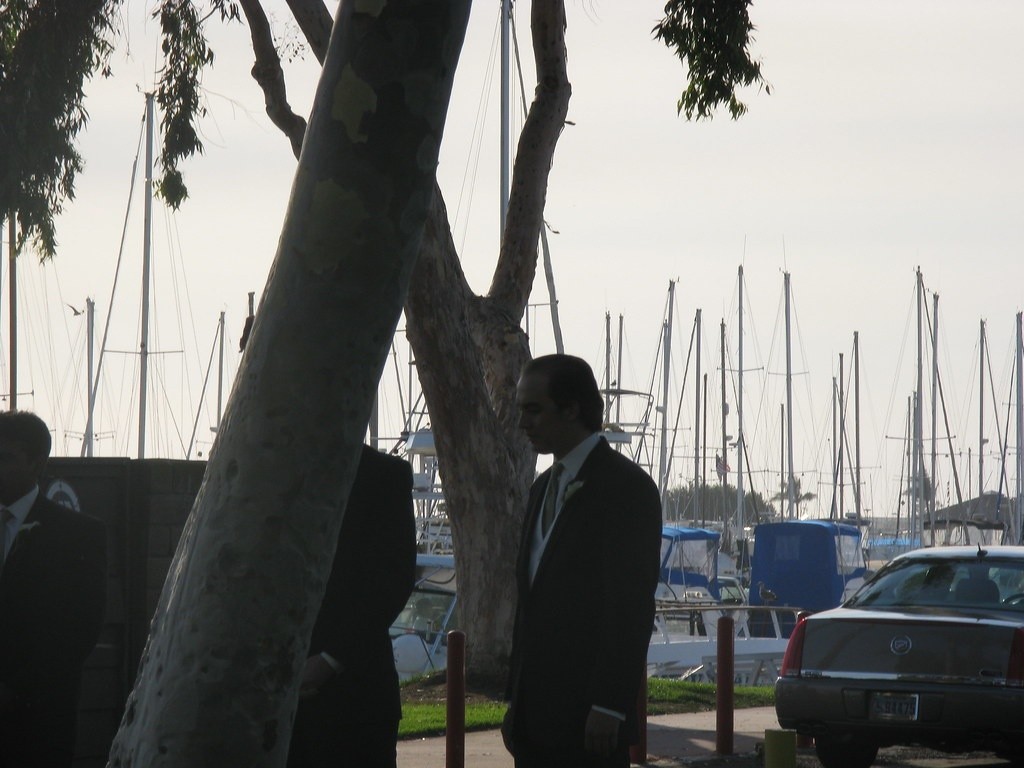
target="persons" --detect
[501,353,663,767]
[0,417,107,768]
[284,440,417,768]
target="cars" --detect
[775,546,1024,768]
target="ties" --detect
[541,462,566,540]
[0,509,14,568]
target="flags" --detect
[715,454,730,482]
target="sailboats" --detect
[2,91,1024,692]
